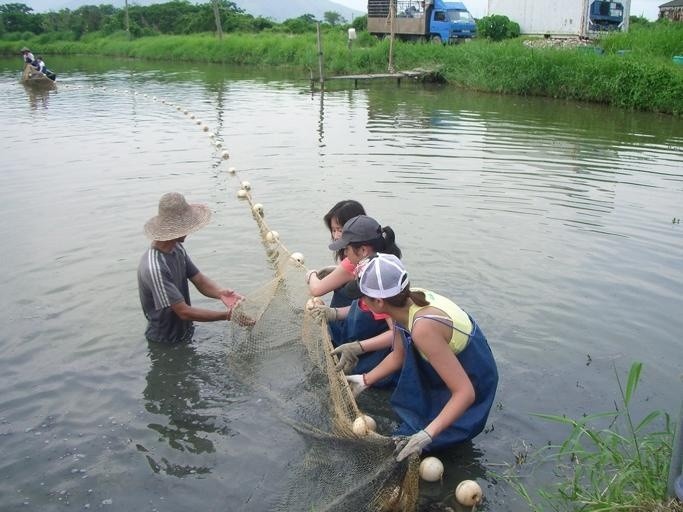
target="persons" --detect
[36,55,46,74]
[21,45,35,65]
[135,193,256,350]
[343,252,499,463]
[304,200,401,387]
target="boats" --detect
[19,72,52,87]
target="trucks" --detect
[366,0,478,48]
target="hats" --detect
[352,252,409,298]
[143,192,213,242]
[329,215,382,251]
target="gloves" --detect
[331,341,365,372]
[308,305,337,322]
[394,429,432,462]
[317,266,336,280]
[344,373,368,400]
[303,269,318,284]
